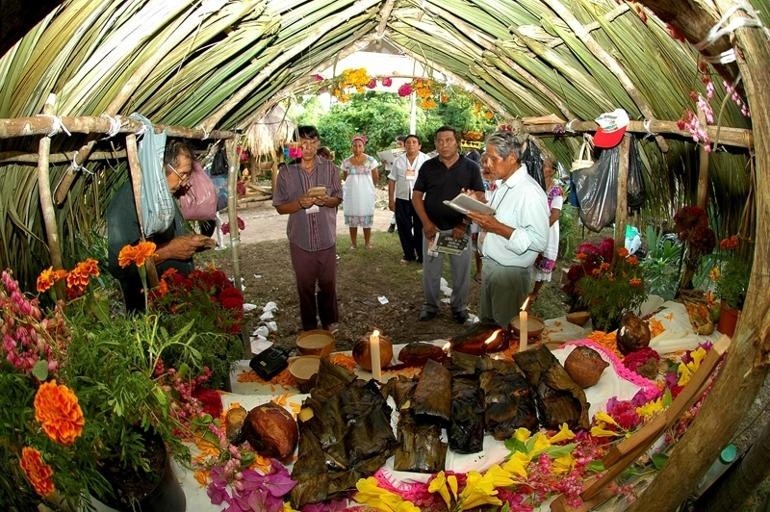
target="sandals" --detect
[329,323,340,338]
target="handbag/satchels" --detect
[570,161,595,207]
[138,130,175,236]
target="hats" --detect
[594,108,629,148]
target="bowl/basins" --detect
[565,311,591,326]
[289,329,336,390]
[509,315,547,340]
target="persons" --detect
[275,125,344,332]
[340,135,380,250]
[288,146,331,165]
[464,130,563,330]
[104,140,216,320]
[411,126,485,324]
[387,135,424,264]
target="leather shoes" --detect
[454,310,469,323]
[419,310,436,321]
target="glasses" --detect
[168,163,190,187]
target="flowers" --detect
[312,67,502,128]
[215,132,251,239]
[617,0,756,158]
